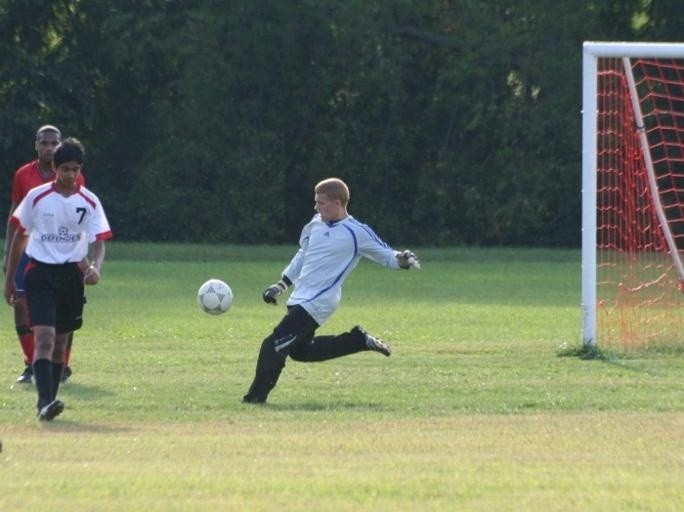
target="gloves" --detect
[262,280,288,305]
[395,250,420,270]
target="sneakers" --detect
[17,367,32,382]
[61,366,72,382]
[36,399,64,422]
[351,324,390,357]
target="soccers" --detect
[198,279,232,314]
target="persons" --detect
[3,124,113,420]
[241,177,419,404]
